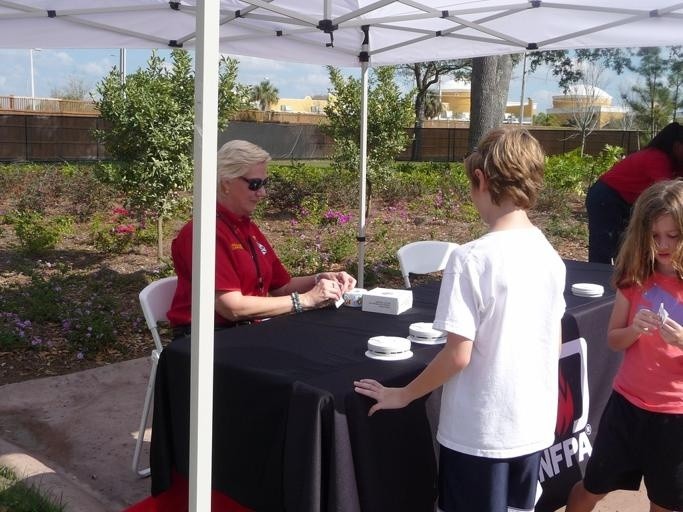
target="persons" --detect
[586,122,683,265]
[353,126,567,512]
[565,177,682,512]
[166,138,358,338]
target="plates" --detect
[365,351,413,360]
[407,335,447,345]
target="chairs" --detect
[132,276,179,478]
[397,240,461,288]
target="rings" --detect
[332,281,336,288]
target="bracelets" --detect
[314,272,321,284]
[290,291,303,315]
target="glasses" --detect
[238,176,268,191]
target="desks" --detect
[151,260,626,512]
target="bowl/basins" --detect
[409,322,447,341]
[368,336,411,355]
